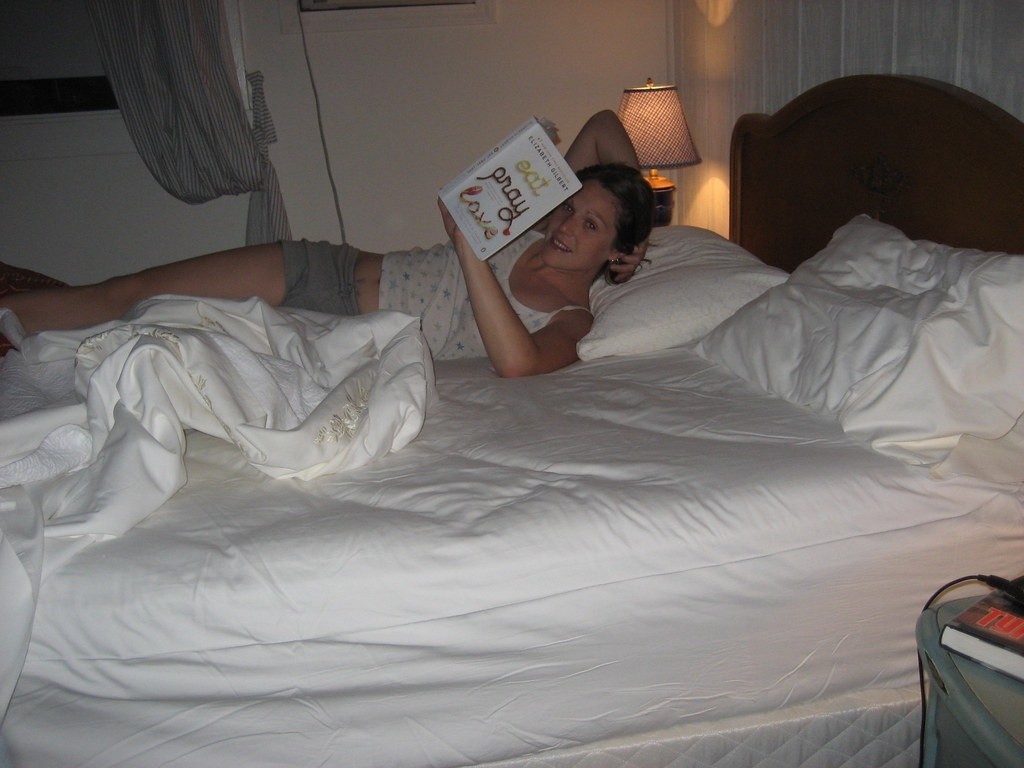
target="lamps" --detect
[618,77,702,228]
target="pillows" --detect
[576,225,787,363]
[690,214,1024,468]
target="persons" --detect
[0,109,661,379]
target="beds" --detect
[0,73,1024,768]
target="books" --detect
[939,577,1024,679]
[438,116,583,262]
